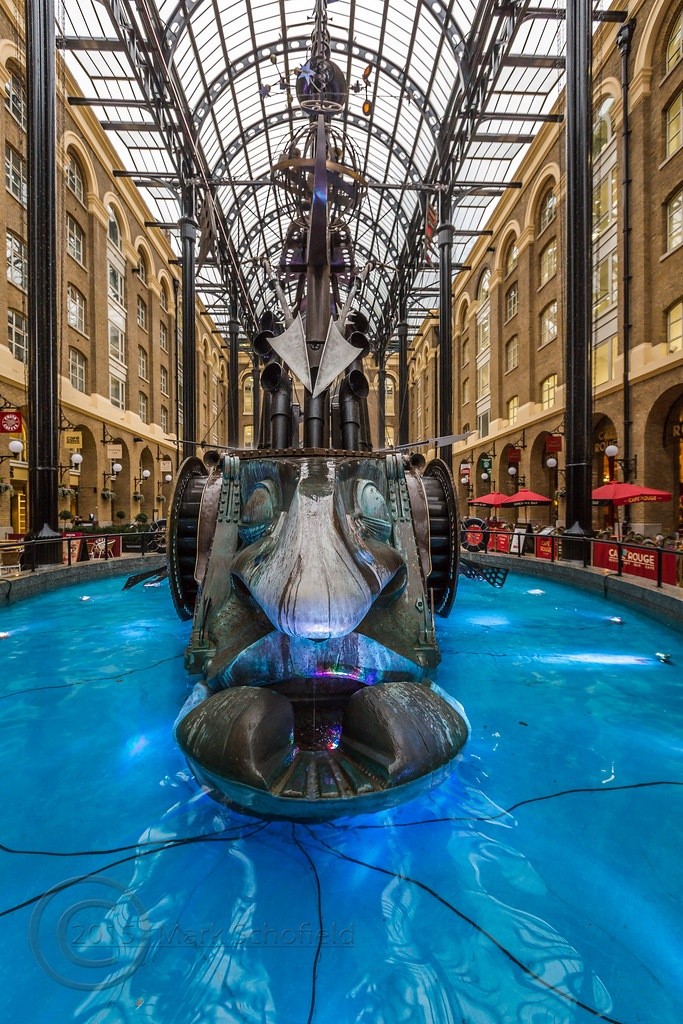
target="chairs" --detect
[626,532,683,553]
[0,540,25,576]
[87,537,116,559]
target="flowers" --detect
[554,488,567,499]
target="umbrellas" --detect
[592,478,672,541]
[467,491,509,517]
[501,488,553,523]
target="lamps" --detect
[481,473,495,491]
[547,458,565,480]
[508,467,525,487]
[605,445,637,479]
[461,478,473,492]
[59,454,83,484]
[157,475,172,492]
[135,470,151,491]
[0,441,23,465]
[103,463,122,488]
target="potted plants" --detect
[101,490,117,500]
[58,487,76,501]
[0,483,16,496]
[132,494,145,503]
[157,495,166,505]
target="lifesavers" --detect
[146,518,166,555]
[459,518,489,552]
